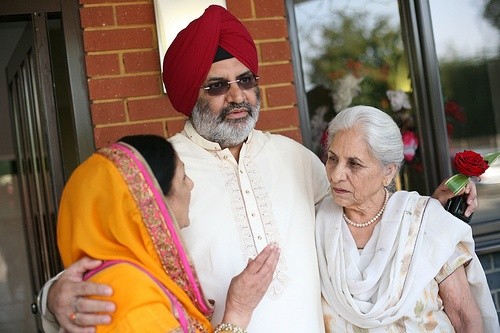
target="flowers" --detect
[452,150,490,177]
[310,59,466,161]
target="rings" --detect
[69,311,80,325]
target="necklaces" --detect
[343,187,389,227]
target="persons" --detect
[58,134,280,333]
[34,5,479,333]
[315,105,500,333]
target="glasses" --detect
[200,74,260,96]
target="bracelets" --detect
[36,286,43,316]
[213,322,247,333]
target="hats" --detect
[163,5,258,117]
